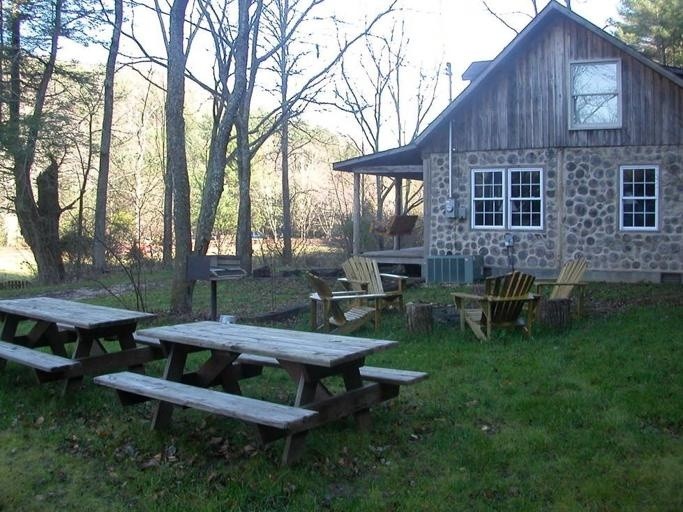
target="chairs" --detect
[307,254,408,336]
[448,256,591,342]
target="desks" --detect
[235,354,429,386]
[138,323,399,465]
[93,370,319,433]
[0,297,161,397]
[1,336,81,383]
